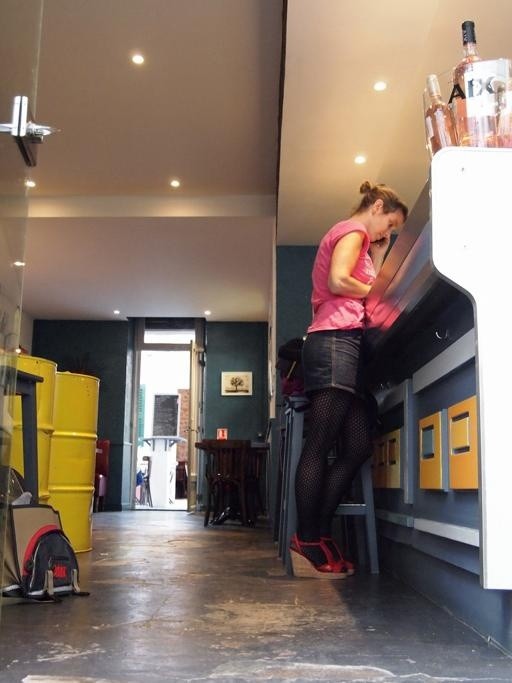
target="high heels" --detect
[289,533,355,580]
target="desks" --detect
[194,439,251,527]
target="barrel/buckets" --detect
[421,59,512,158]
[48,371,101,553]
[0,351,59,506]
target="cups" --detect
[461,58,512,148]
[216,427,228,440]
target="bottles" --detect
[452,20,484,148]
[424,73,456,156]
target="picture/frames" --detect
[221,371,254,396]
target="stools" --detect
[276,393,385,575]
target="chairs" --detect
[202,440,247,526]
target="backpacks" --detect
[22,525,89,605]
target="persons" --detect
[286,180,410,580]
